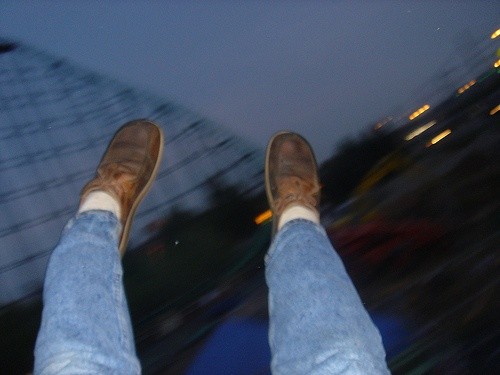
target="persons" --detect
[32,117,393,375]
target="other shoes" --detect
[263,131,323,248]
[78,116,162,260]
